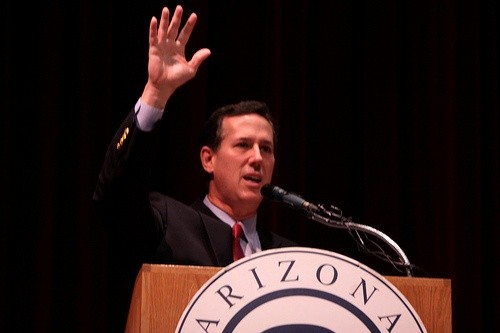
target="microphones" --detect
[260,184,320,213]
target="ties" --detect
[232,223,245,262]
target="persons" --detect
[103,5,304,267]
